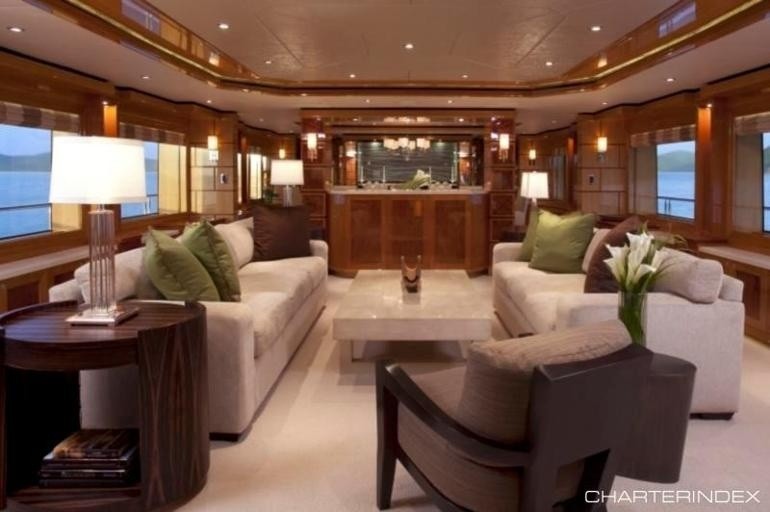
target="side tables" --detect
[496,225,528,242]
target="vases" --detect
[617,289,648,350]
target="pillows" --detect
[73,215,255,303]
[580,217,724,303]
[250,200,314,262]
[515,204,595,274]
[452,316,634,469]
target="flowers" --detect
[601,215,690,348]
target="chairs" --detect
[375,343,699,512]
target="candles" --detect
[428,166,432,180]
[451,166,453,180]
[360,167,363,181]
[382,165,386,181]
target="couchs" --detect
[48,238,330,442]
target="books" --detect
[36,428,140,489]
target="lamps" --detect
[498,132,510,163]
[596,136,608,161]
[207,135,220,161]
[383,115,432,152]
[306,132,318,163]
[520,170,550,206]
[269,157,305,208]
[47,134,150,328]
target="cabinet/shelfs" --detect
[700,251,770,346]
[0,298,212,512]
[323,180,492,278]
